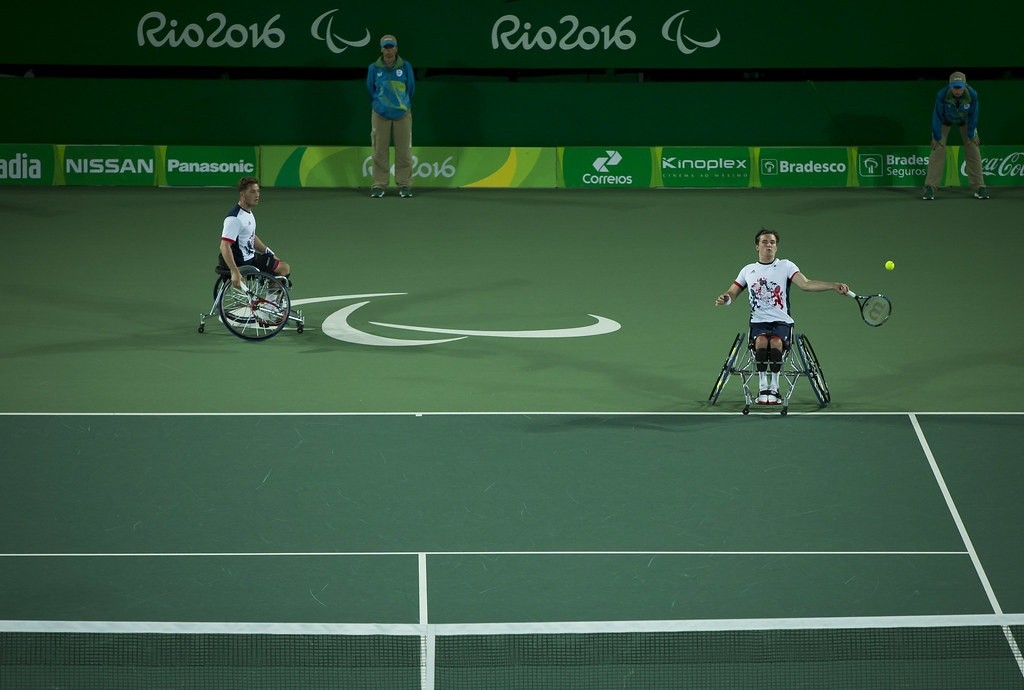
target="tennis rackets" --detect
[839,284,893,327]
[238,281,281,325]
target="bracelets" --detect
[264,247,275,256]
[725,294,731,305]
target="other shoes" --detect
[264,300,282,312]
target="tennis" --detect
[886,261,895,271]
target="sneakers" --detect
[399,186,412,197]
[975,187,990,199]
[922,185,935,200]
[371,188,385,198]
[768,384,779,405]
[759,384,768,405]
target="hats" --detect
[949,72,966,88]
[380,35,397,48]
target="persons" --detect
[218,177,290,312]
[366,34,415,198]
[922,72,990,200]
[715,229,849,406]
